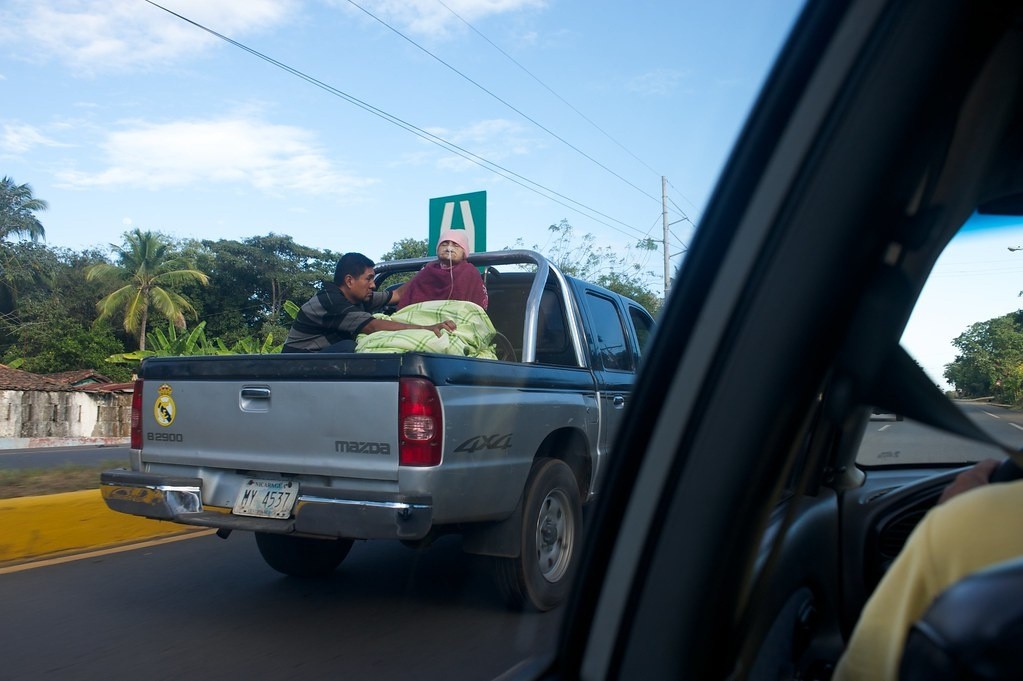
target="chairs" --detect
[899,558,1023,681]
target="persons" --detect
[397,230,488,312]
[281,252,457,353]
[832,458,1023,681]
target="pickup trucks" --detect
[95,247,658,614]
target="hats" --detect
[436,229,469,260]
